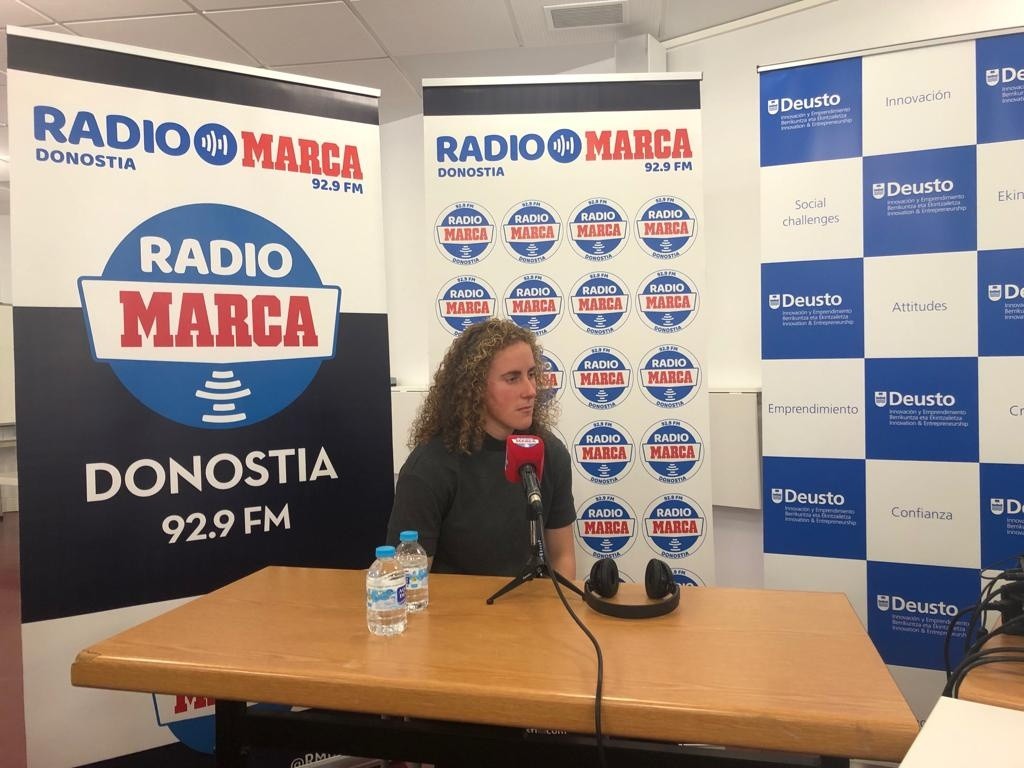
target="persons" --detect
[387,317,579,581]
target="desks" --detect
[898,696,1024,768]
[958,613,1024,712]
[73,567,921,768]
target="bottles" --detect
[366,545,408,635]
[394,530,429,613]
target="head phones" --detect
[583,556,681,617]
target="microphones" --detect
[504,435,544,502]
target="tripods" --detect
[485,510,586,601]
